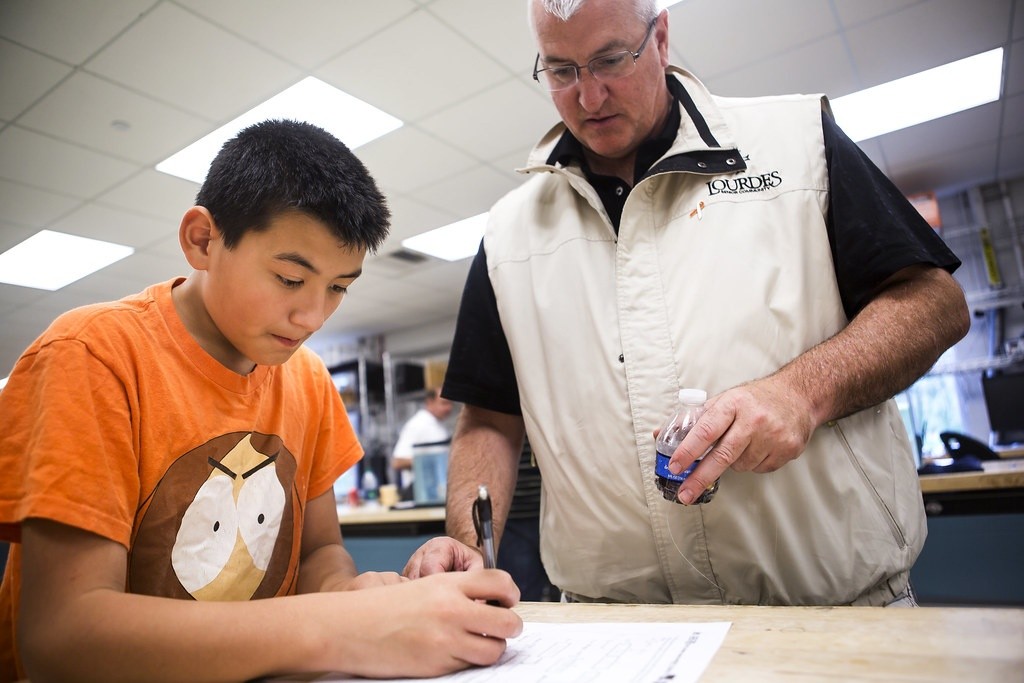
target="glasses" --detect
[532,16,656,92]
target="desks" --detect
[251,600,1024,683]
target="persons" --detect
[391,387,454,502]
[0,122,522,683]
[401,0,969,608]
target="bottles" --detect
[362,467,379,505]
[655,389,720,506]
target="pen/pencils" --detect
[470,486,501,644]
